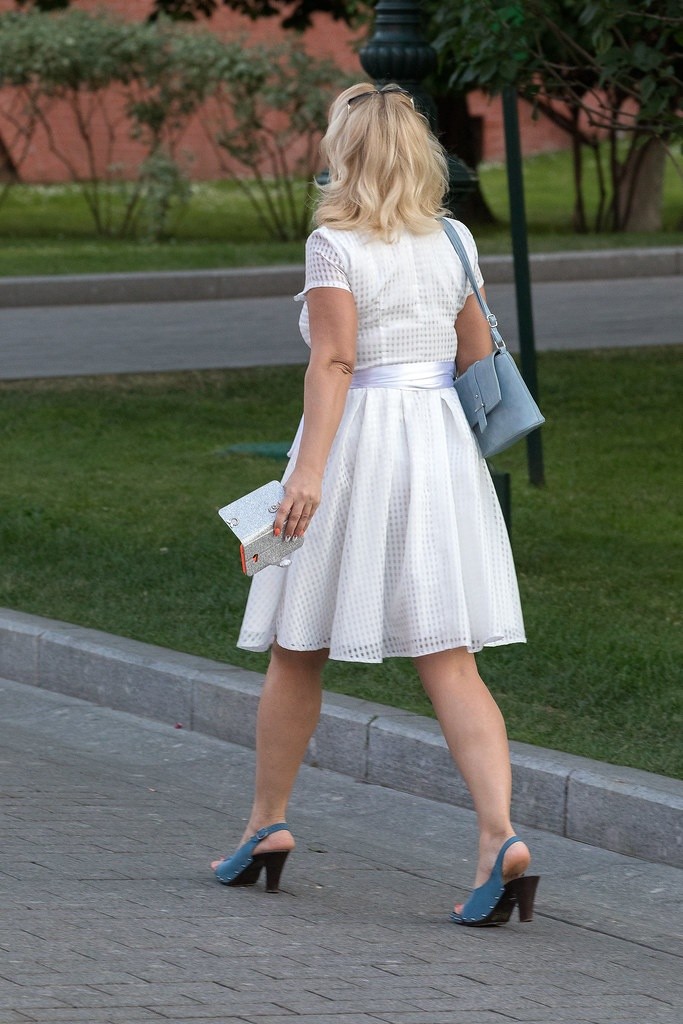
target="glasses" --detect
[347,87,412,107]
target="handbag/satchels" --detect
[436,217,545,458]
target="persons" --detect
[210,82,539,926]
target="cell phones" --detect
[239,519,304,576]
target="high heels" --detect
[212,823,291,893]
[449,836,541,927]
